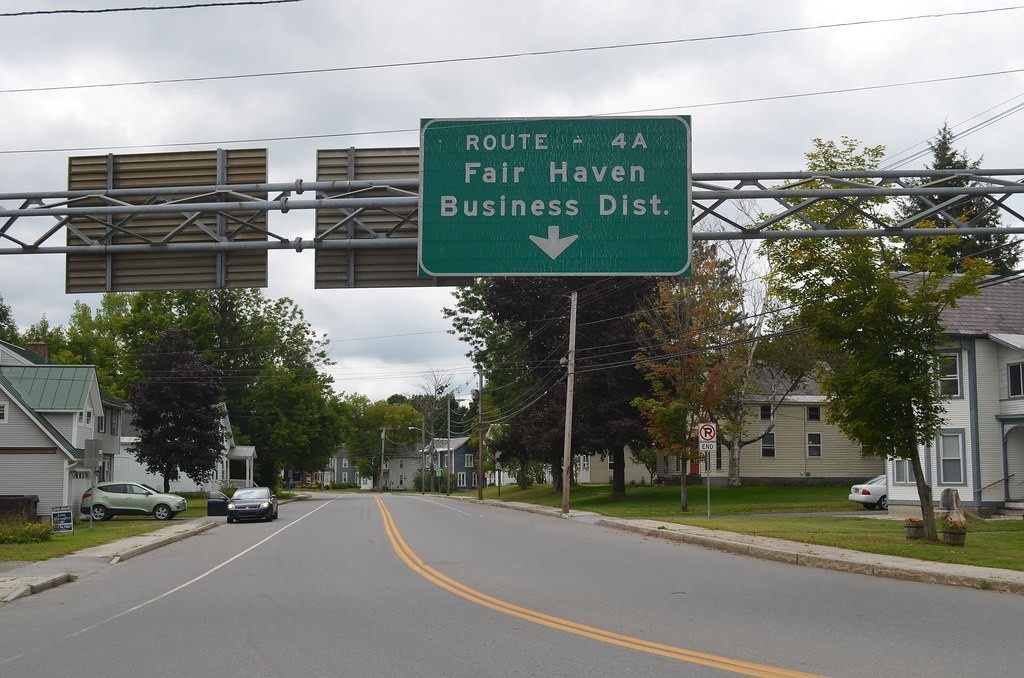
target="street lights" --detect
[447,399,465,496]
[408,426,425,496]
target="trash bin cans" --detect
[0,494,40,520]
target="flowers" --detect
[941,517,966,530]
[904,515,924,525]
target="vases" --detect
[904,524,924,540]
[942,528,966,547]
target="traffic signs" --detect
[417,116,693,278]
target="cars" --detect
[207,487,279,524]
[81,482,188,521]
[848,475,888,511]
